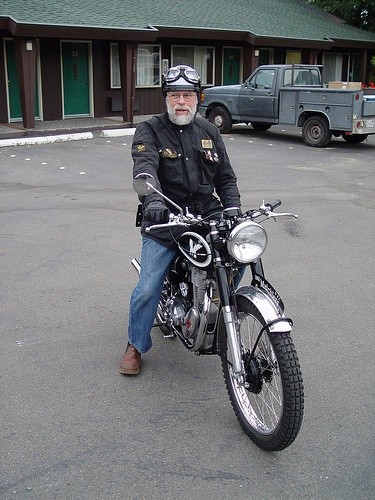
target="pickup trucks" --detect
[199,61,375,149]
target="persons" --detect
[121,66,242,375]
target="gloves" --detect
[144,201,169,224]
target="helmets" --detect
[162,65,202,95]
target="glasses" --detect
[163,67,201,84]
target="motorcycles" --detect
[130,170,307,452]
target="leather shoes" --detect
[119,341,141,374]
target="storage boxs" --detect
[362,95,375,117]
[329,81,362,90]
